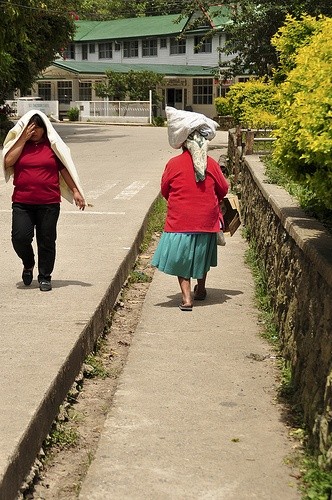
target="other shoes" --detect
[39,279,52,291]
[22,265,33,286]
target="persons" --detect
[150,107,229,311]
[2,109,86,292]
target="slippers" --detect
[193,284,206,300]
[179,302,192,311]
